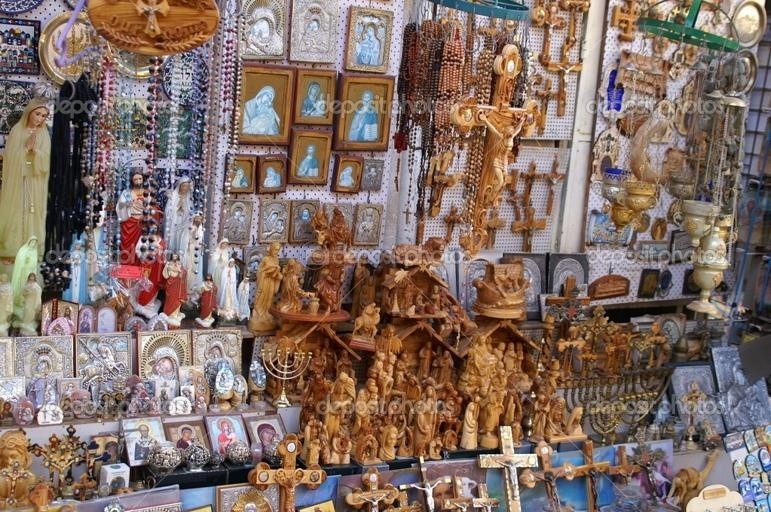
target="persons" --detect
[0,24,585,507]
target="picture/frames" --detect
[0,328,288,467]
